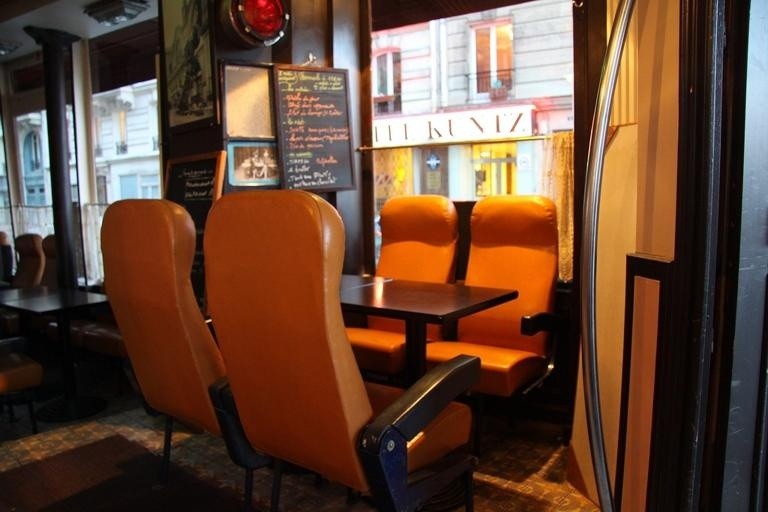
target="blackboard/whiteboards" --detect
[273,63,356,192]
[164,150,227,318]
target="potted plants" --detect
[489,80,508,103]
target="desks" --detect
[341,274,518,387]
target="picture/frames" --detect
[217,57,277,140]
[158,1,220,137]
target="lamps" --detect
[82,1,151,26]
[0,40,22,56]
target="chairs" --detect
[101,196,254,510]
[423,195,560,430]
[346,195,460,387]
[198,187,481,509]
